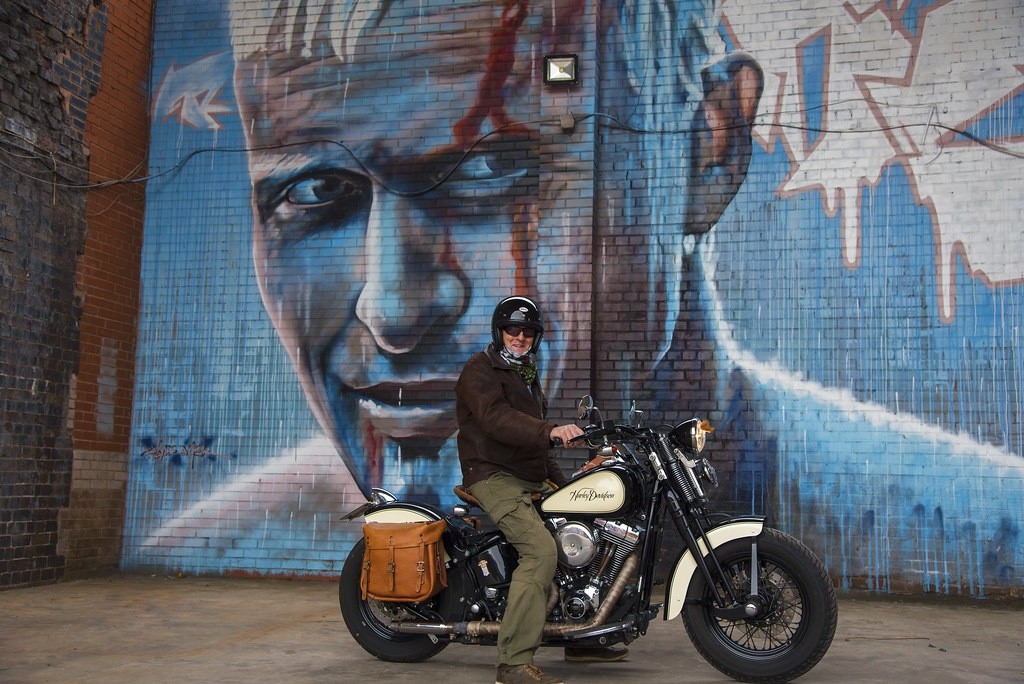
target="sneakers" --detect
[495,663,564,684]
[564,646,630,661]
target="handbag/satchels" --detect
[361,520,448,602]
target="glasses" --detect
[501,325,536,338]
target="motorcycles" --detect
[338,395,838,683]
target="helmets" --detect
[490,295,545,355]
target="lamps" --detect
[542,54,579,86]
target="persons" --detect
[454,294,631,684]
